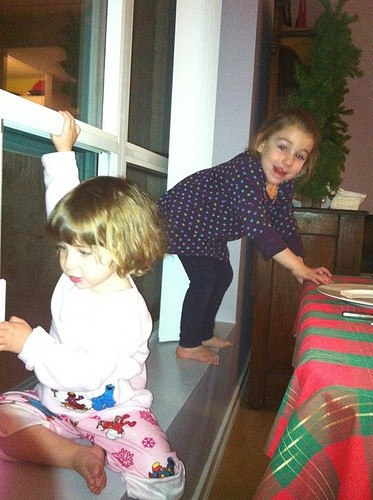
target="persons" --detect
[1,109,188,500]
[153,105,337,366]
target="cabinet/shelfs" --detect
[249,208,366,412]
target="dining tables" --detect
[252,275,373,499]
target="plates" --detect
[317,284,373,308]
